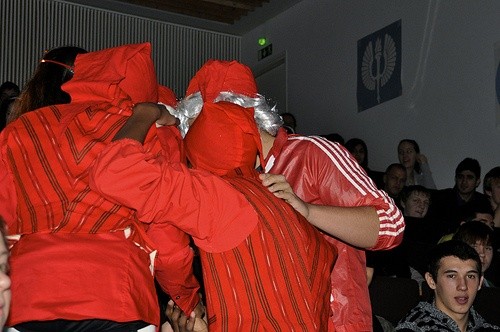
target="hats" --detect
[57,40,160,144]
[180,100,265,173]
[184,58,259,103]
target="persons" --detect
[0,95,18,133]
[280,113,297,135]
[0,41,202,331]
[6,47,89,123]
[89,100,339,332]
[394,239,500,332]
[318,133,499,290]
[182,58,406,331]
[0,81,19,106]
